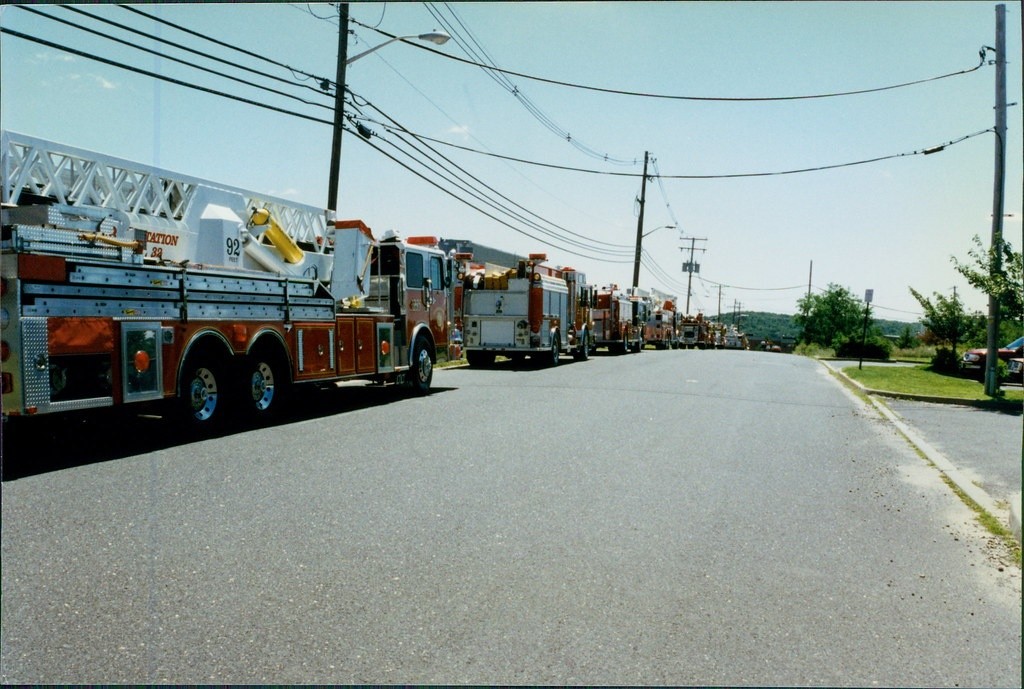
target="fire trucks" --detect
[1,128,458,442]
[592,280,751,354]
[458,251,598,368]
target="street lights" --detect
[327,32,450,213]
[632,225,677,288]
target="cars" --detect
[771,345,782,352]
[958,335,1024,386]
